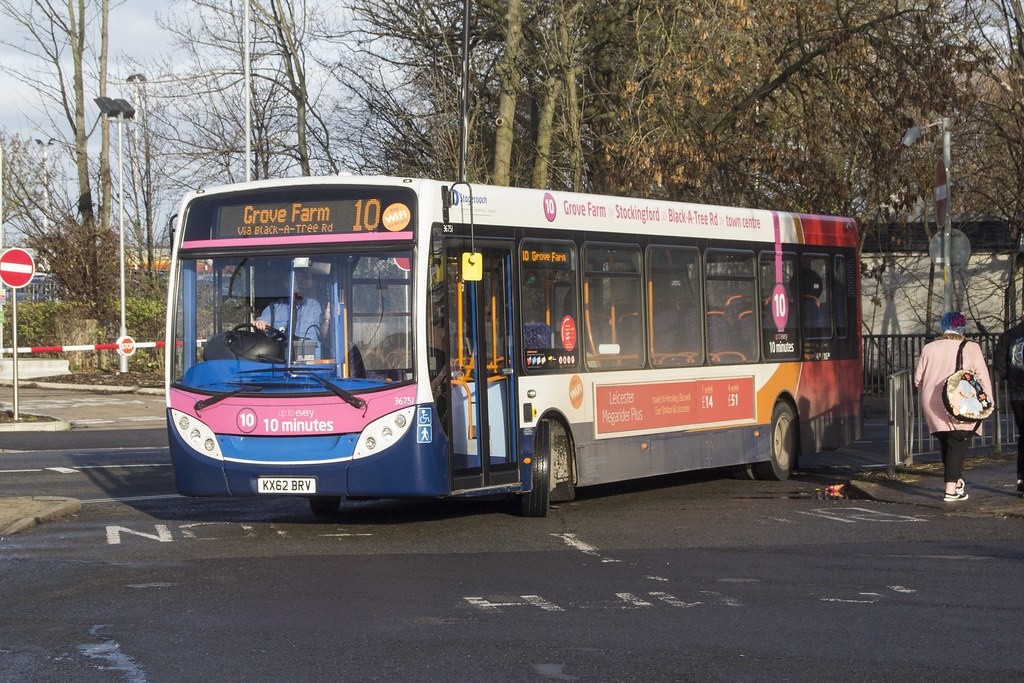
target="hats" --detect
[941,312,968,331]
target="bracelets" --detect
[324,318,330,322]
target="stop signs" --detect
[0,248,35,289]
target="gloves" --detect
[253,320,271,330]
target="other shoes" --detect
[1018,479,1024,492]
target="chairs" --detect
[591,294,846,365]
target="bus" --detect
[163,169,868,517]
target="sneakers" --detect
[943,493,969,501]
[955,478,965,495]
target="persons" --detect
[993,319,1024,492]
[252,268,341,359]
[914,313,995,501]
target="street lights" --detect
[899,116,953,314]
[35,138,57,252]
[93,95,136,373]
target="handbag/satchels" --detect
[942,370,994,422]
